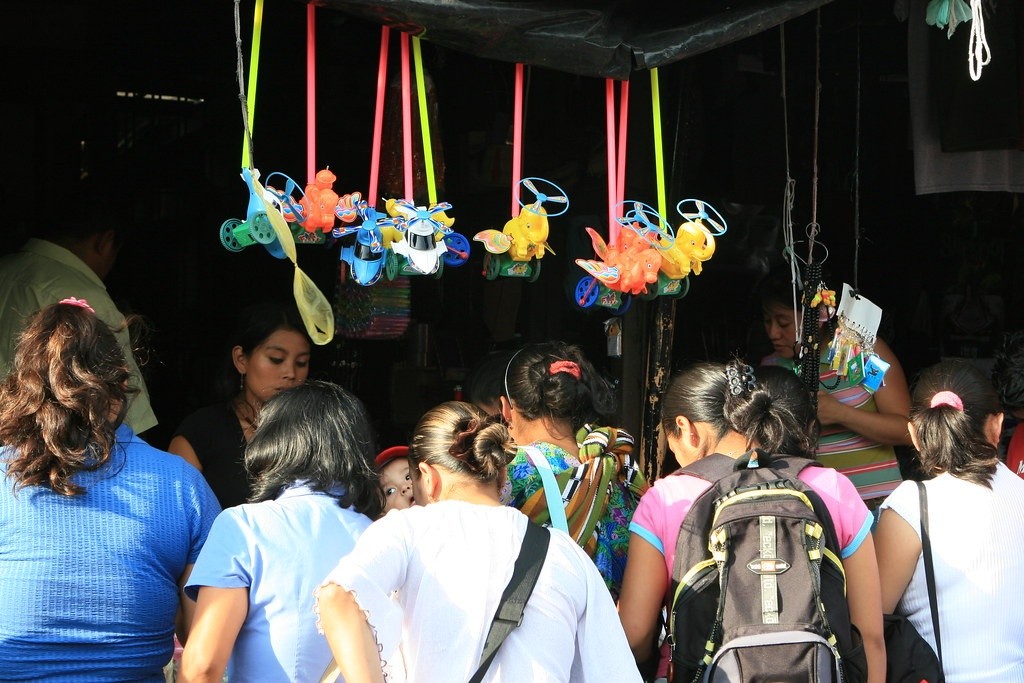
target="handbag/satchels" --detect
[883,479,944,683]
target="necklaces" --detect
[233,402,257,430]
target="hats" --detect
[374,446,409,473]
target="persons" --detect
[0,192,1024,683]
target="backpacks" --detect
[670,447,869,683]
